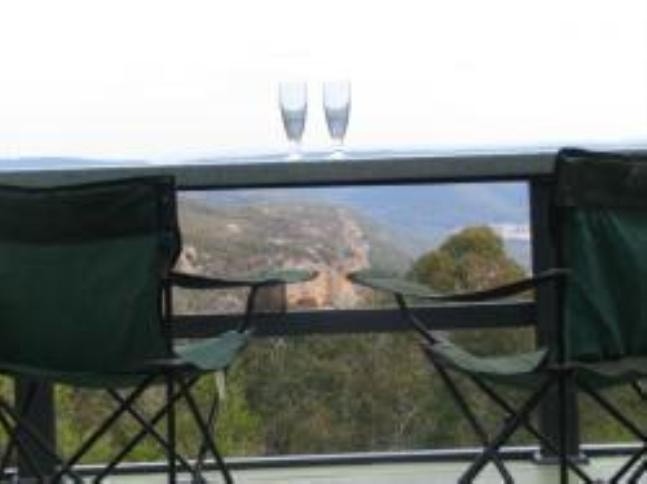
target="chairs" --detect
[344,146,647,484]
[1,171,319,484]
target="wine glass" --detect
[276,82,308,160]
[321,77,353,160]
